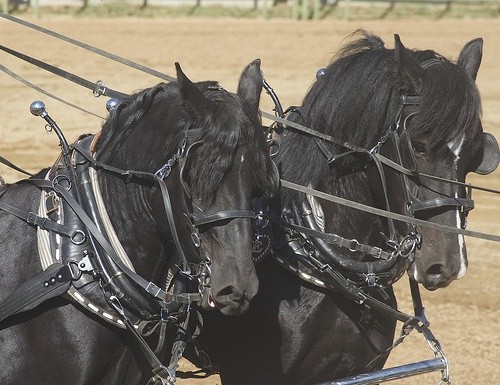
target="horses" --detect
[177,26,487,385]
[0,59,280,384]
